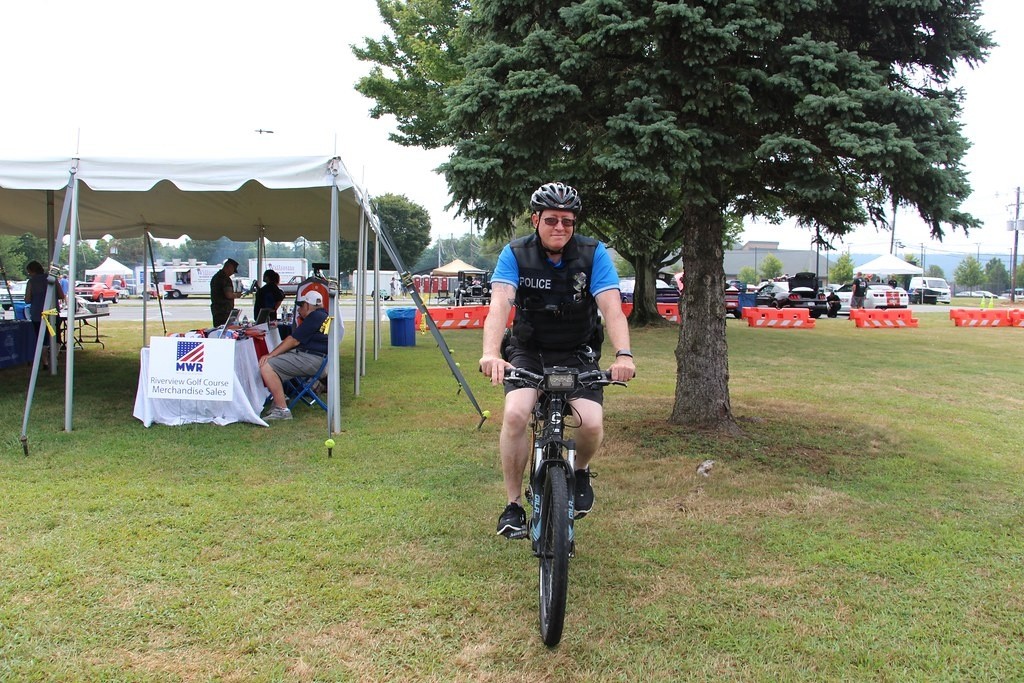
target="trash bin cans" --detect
[389,308,418,347]
[737,292,755,318]
[13,301,28,320]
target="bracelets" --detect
[616,349,633,359]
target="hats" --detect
[297,291,323,306]
[467,276,473,280]
[228,258,239,273]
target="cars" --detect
[954,291,999,299]
[909,277,950,304]
[618,277,678,303]
[453,270,490,307]
[830,280,909,317]
[276,275,338,297]
[75,282,121,303]
[0,280,29,310]
[753,281,829,319]
[672,273,739,317]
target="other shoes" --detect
[43,364,48,370]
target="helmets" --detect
[529,182,582,214]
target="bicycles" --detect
[479,353,629,649]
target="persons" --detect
[60,275,69,309]
[120,278,126,288]
[847,272,868,320]
[864,275,898,289]
[24,261,65,370]
[210,259,244,328]
[827,292,841,318]
[259,290,330,421]
[622,296,629,303]
[390,277,396,301]
[254,269,279,325]
[479,182,636,535]
[472,277,482,285]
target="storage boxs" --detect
[88,302,109,314]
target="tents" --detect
[852,252,924,304]
[429,259,486,306]
[0,154,491,458]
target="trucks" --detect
[134,258,243,299]
[352,269,402,301]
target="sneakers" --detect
[262,405,293,419]
[266,394,289,415]
[574,455,598,513]
[496,502,527,536]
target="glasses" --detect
[540,217,576,226]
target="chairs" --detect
[287,355,328,410]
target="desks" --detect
[133,328,281,427]
[58,311,110,350]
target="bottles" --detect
[0,308,5,325]
[243,315,247,324]
